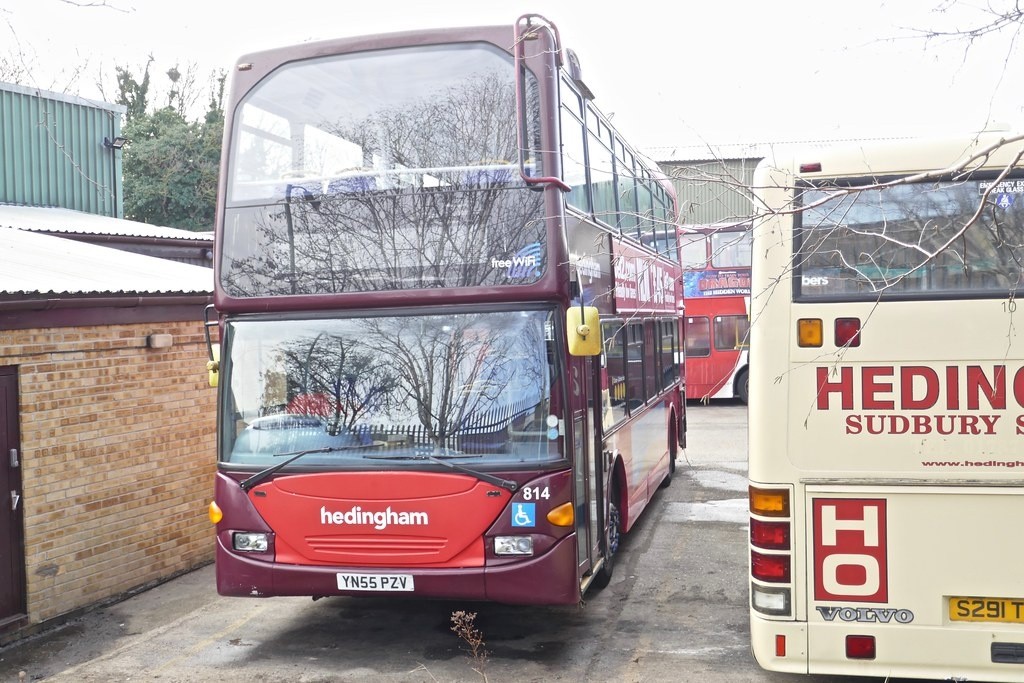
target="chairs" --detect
[255,160,540,278]
[283,317,561,458]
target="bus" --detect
[740,137,1024,683]
[667,216,749,406]
[202,16,686,615]
